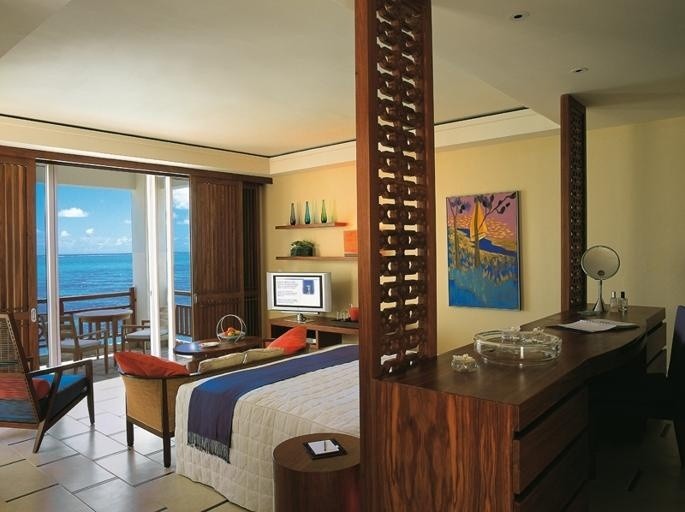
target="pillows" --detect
[197,350,244,374]
[114,350,191,379]
[244,344,284,362]
[265,325,309,355]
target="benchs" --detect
[118,336,310,470]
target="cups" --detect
[348,307,358,320]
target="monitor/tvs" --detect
[266,272,332,323]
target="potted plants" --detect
[291,240,315,257]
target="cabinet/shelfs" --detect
[273,222,348,263]
[266,315,360,349]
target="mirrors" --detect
[581,244,622,314]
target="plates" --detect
[199,342,220,347]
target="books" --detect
[545,317,640,332]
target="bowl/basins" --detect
[474,329,564,367]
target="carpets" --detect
[63,356,120,384]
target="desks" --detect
[273,430,361,512]
[75,308,134,372]
[368,301,668,510]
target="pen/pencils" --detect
[324,440,326,451]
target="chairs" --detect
[589,304,684,480]
[0,307,96,455]
[120,304,178,355]
[59,311,100,375]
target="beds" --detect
[173,343,441,510]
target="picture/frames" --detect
[303,437,347,460]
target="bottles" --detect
[320,198,327,223]
[610,291,618,312]
[450,354,477,373]
[303,201,311,225]
[290,202,297,226]
[619,292,628,311]
